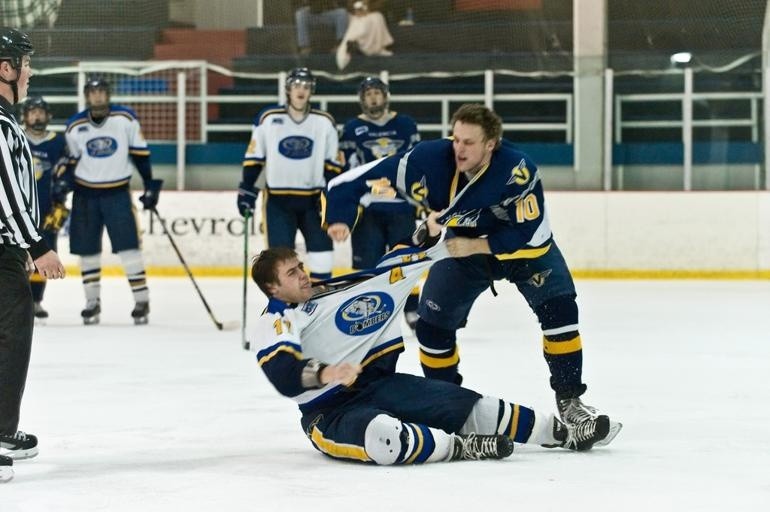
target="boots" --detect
[557,396,624,448]
[34,301,49,326]
[443,432,515,462]
[131,300,150,324]
[81,297,102,325]
[540,414,611,453]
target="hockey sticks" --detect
[150,205,222,331]
[242,207,250,351]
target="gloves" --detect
[236,182,261,218]
[51,178,69,204]
[139,179,163,210]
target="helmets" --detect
[0,26,35,60]
[20,96,51,112]
[358,77,387,92]
[286,66,317,87]
[84,78,111,92]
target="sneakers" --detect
[0,430,39,460]
[0,454,15,482]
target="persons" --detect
[295,0,349,57]
[250,211,609,465]
[0,25,66,483]
[23,97,68,319]
[335,1,394,71]
[237,67,347,255]
[55,79,163,324]
[337,77,423,328]
[322,102,623,446]
